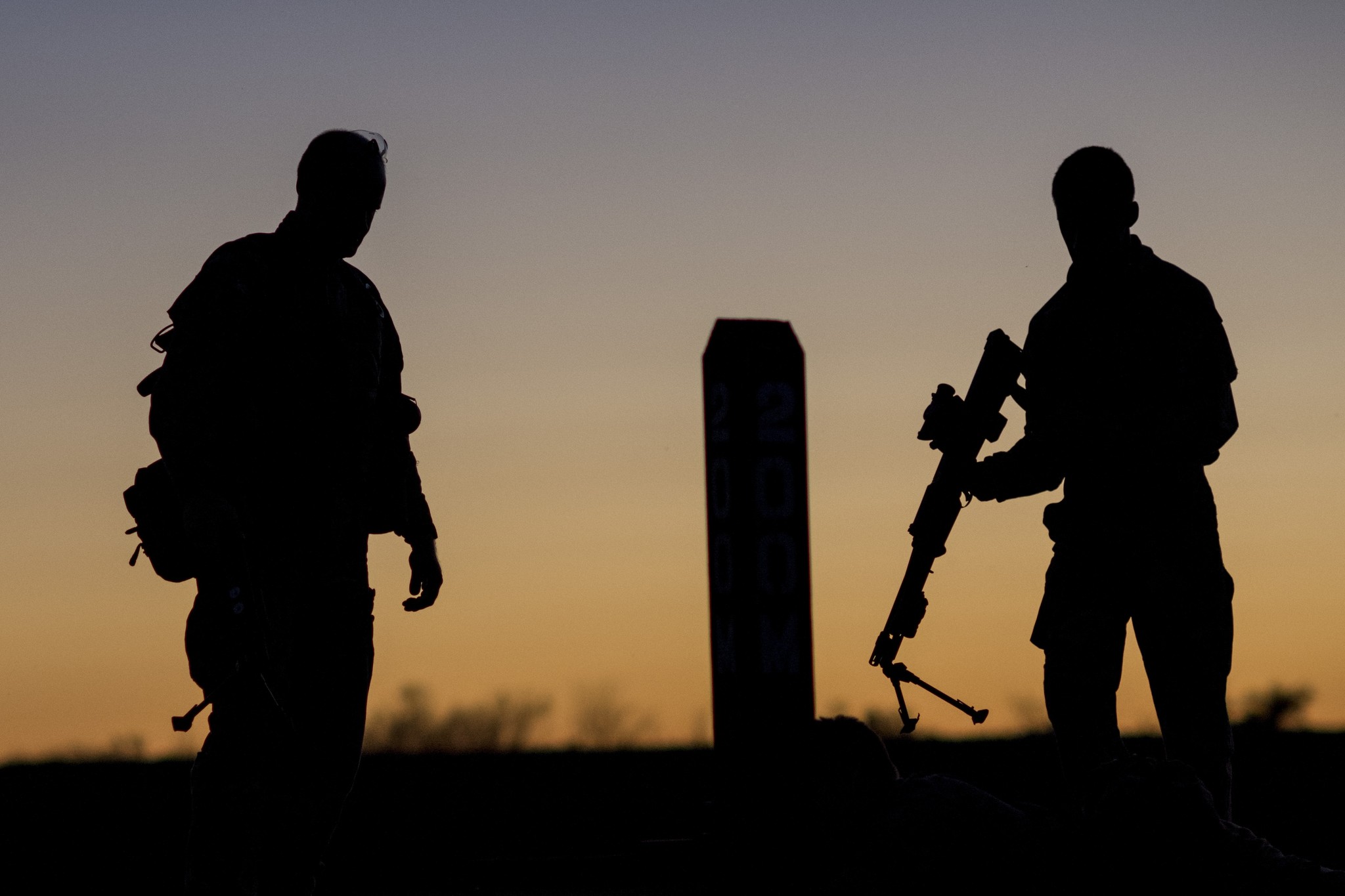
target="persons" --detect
[960,145,1241,895]
[137,127,445,896]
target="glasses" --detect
[350,130,388,164]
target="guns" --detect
[867,329,1028,737]
